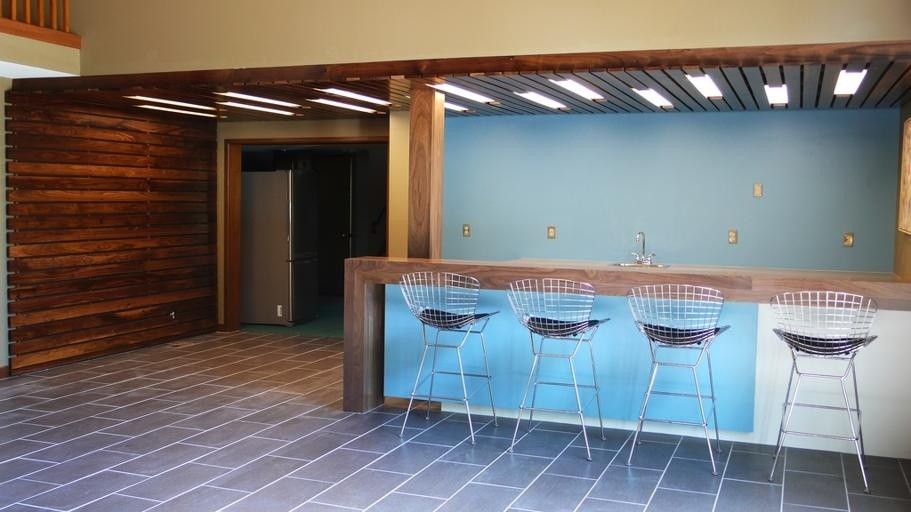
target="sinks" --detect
[611,264,671,269]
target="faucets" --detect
[635,231,645,257]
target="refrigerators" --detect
[241,168,318,325]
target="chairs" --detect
[398,286,500,445]
[623,283,731,476]
[767,289,879,495]
[503,276,610,461]
